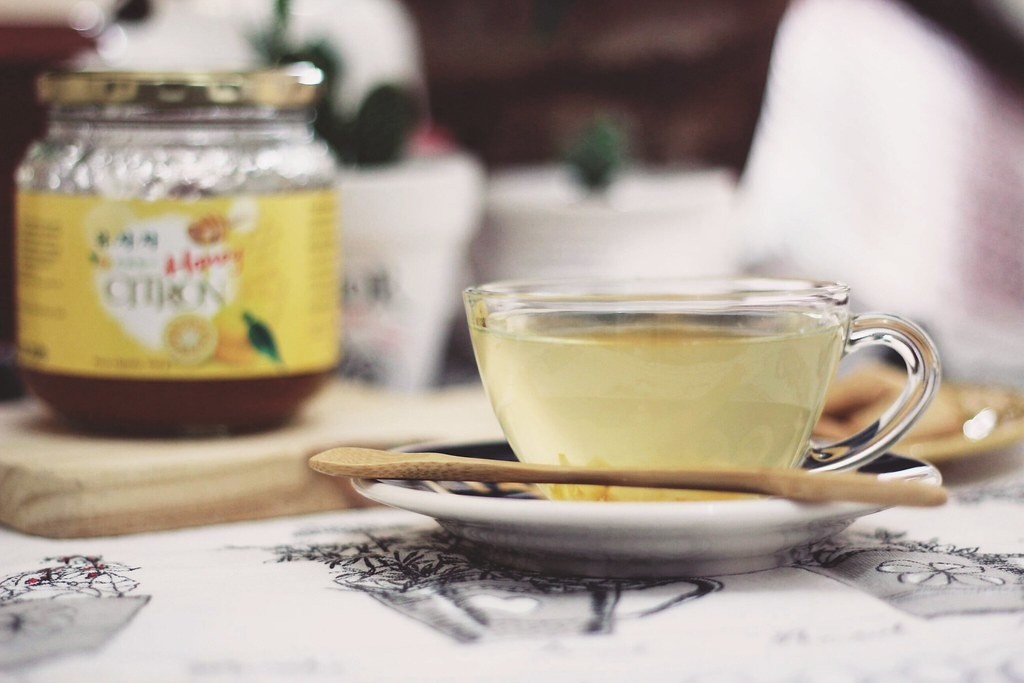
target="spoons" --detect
[309,447,950,509]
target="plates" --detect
[349,437,943,579]
[821,367,1024,460]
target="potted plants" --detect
[235,0,484,391]
[483,126,739,283]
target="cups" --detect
[461,279,941,502]
